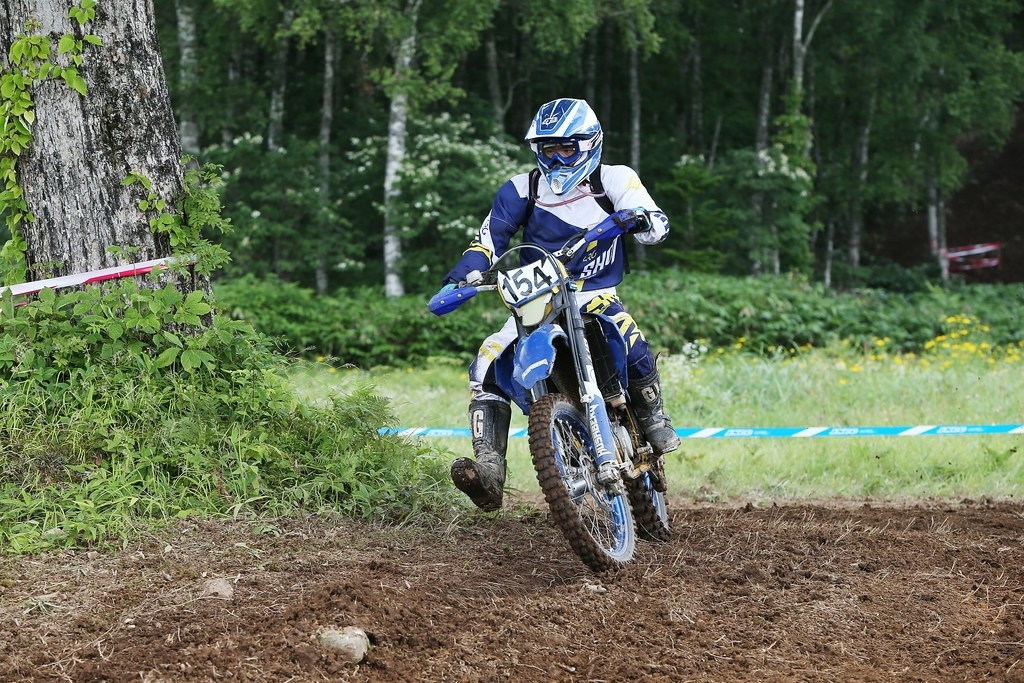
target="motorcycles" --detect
[425,208,671,572]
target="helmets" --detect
[525,98,603,195]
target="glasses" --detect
[535,139,583,170]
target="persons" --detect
[424,96,682,510]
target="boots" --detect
[627,368,681,454]
[451,400,512,511]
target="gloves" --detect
[627,207,653,232]
[438,283,459,292]
